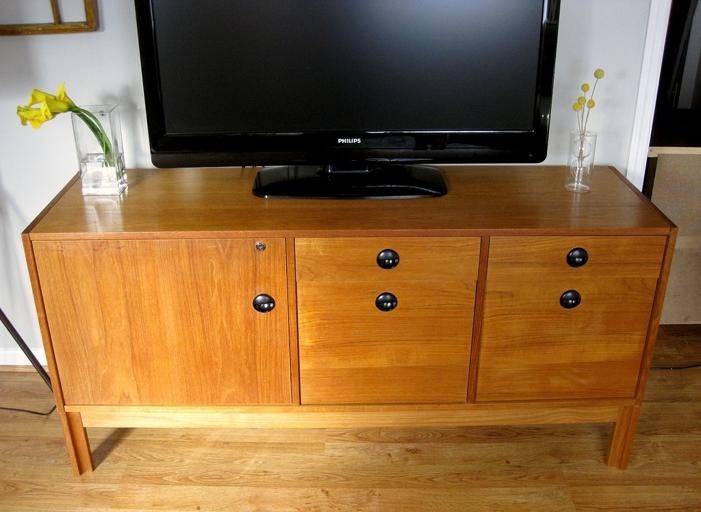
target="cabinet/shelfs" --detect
[21,228,679,427]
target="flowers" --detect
[573,69,605,151]
[17,86,114,165]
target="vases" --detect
[566,129,595,193]
[71,106,126,197]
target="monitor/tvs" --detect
[134,0,561,201]
